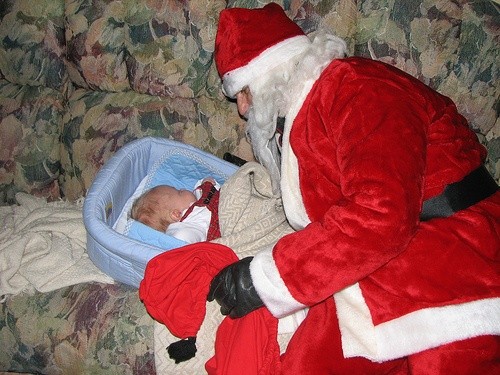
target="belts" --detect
[420,166,498,222]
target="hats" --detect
[214,2,311,99]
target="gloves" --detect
[206,256,265,319]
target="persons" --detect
[130,177,222,244]
[206,2,500,375]
